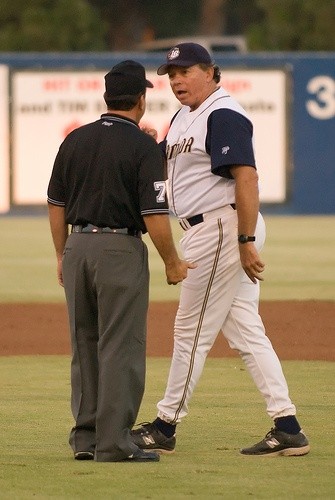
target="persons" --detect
[129,42,310,457]
[46,57,198,463]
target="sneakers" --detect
[242,427,310,457]
[120,446,159,462]
[75,451,93,458]
[129,422,176,454]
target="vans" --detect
[150,34,246,52]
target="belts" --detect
[71,222,142,238]
[179,204,236,231]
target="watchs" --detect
[237,234,256,243]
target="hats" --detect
[157,43,211,75]
[104,61,154,96]
